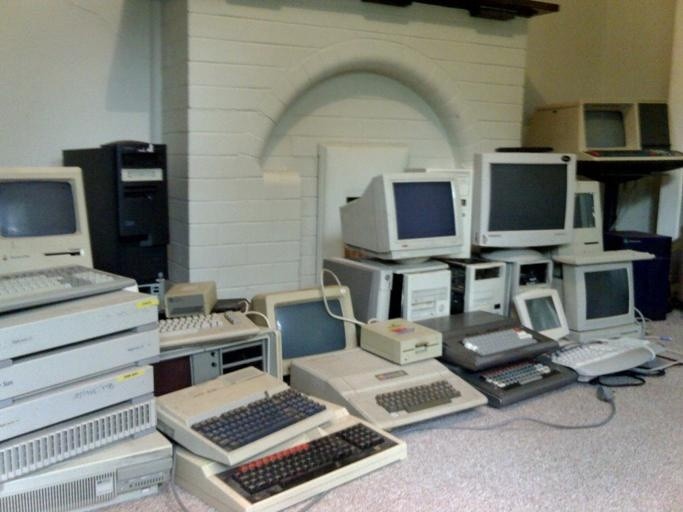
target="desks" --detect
[576,159,681,234]
